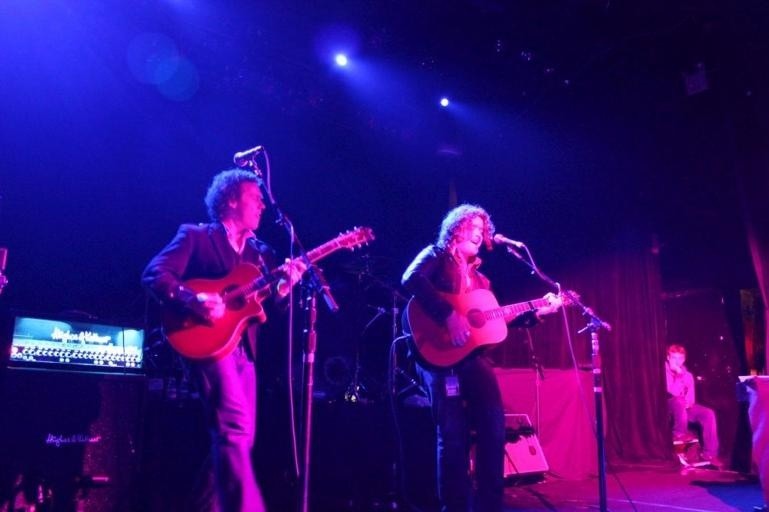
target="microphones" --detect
[394,365,428,396]
[233,145,263,166]
[494,233,523,249]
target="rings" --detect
[464,330,470,336]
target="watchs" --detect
[193,292,207,304]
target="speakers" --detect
[470,413,550,478]
[4,364,148,510]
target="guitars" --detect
[401,290,581,371]
[161,225,376,363]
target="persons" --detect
[141,170,307,512]
[663,343,723,468]
[401,204,564,512]
